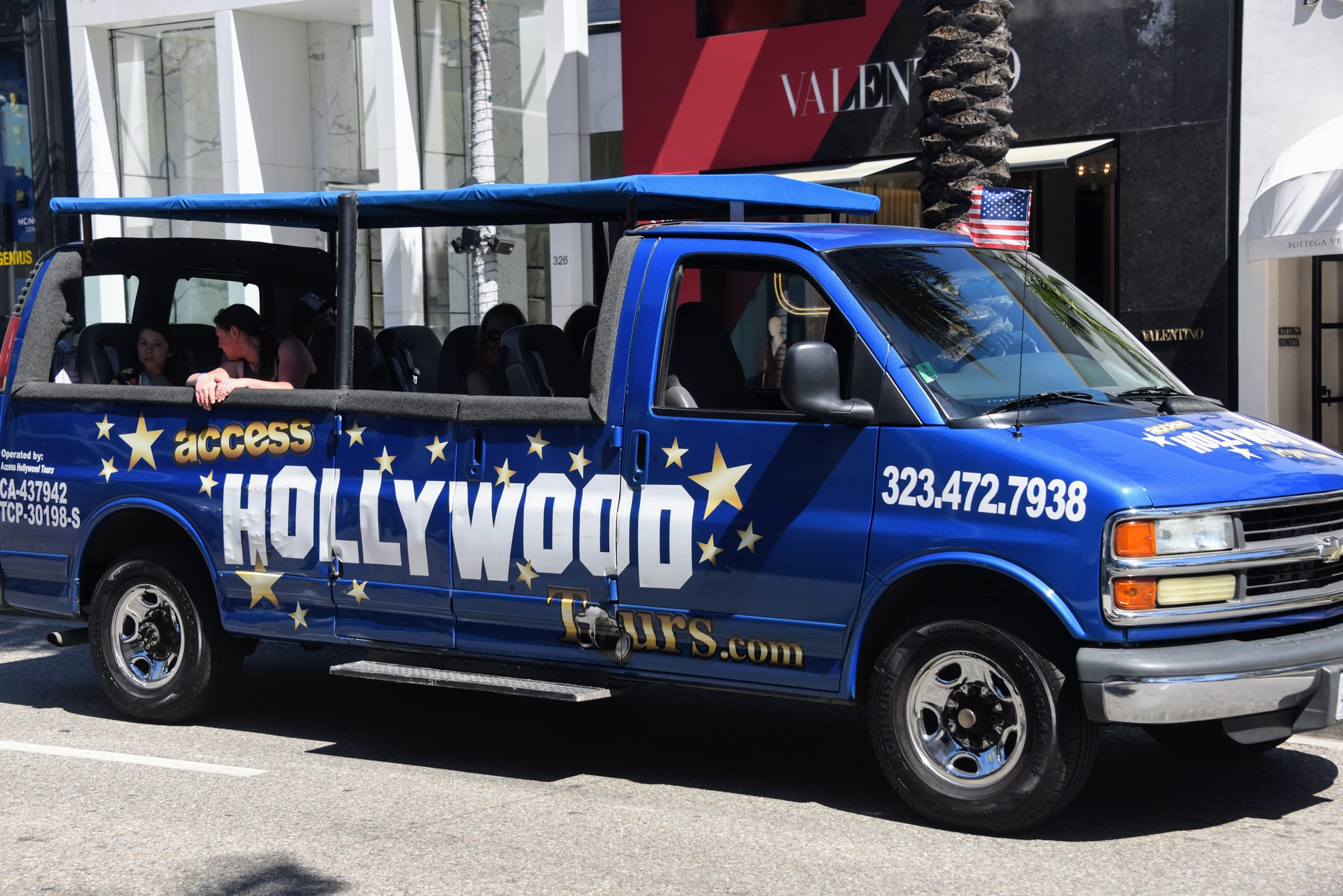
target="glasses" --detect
[487,328,503,343]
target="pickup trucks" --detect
[0,173,1343,837]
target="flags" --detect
[968,186,1032,250]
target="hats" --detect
[298,293,338,315]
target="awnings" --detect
[1005,138,1115,173]
[754,157,917,188]
[1246,116,1343,264]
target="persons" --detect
[291,292,337,346]
[467,303,526,396]
[186,304,324,411]
[110,322,187,387]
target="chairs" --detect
[77,325,876,418]
